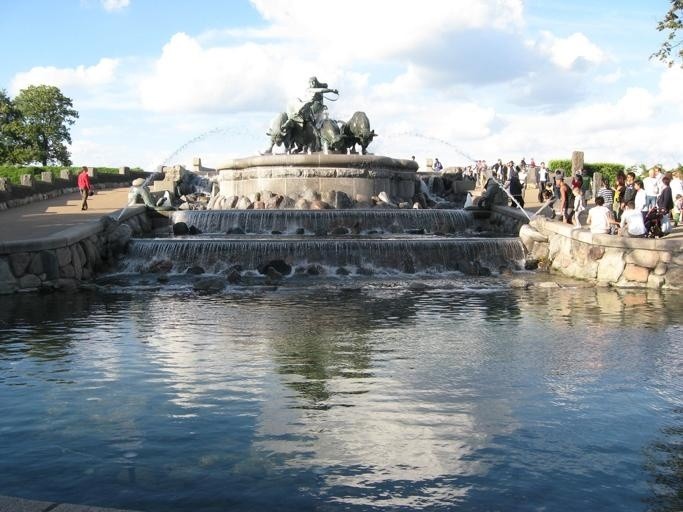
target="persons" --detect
[410,150,683,242]
[294,77,340,107]
[77,167,94,212]
